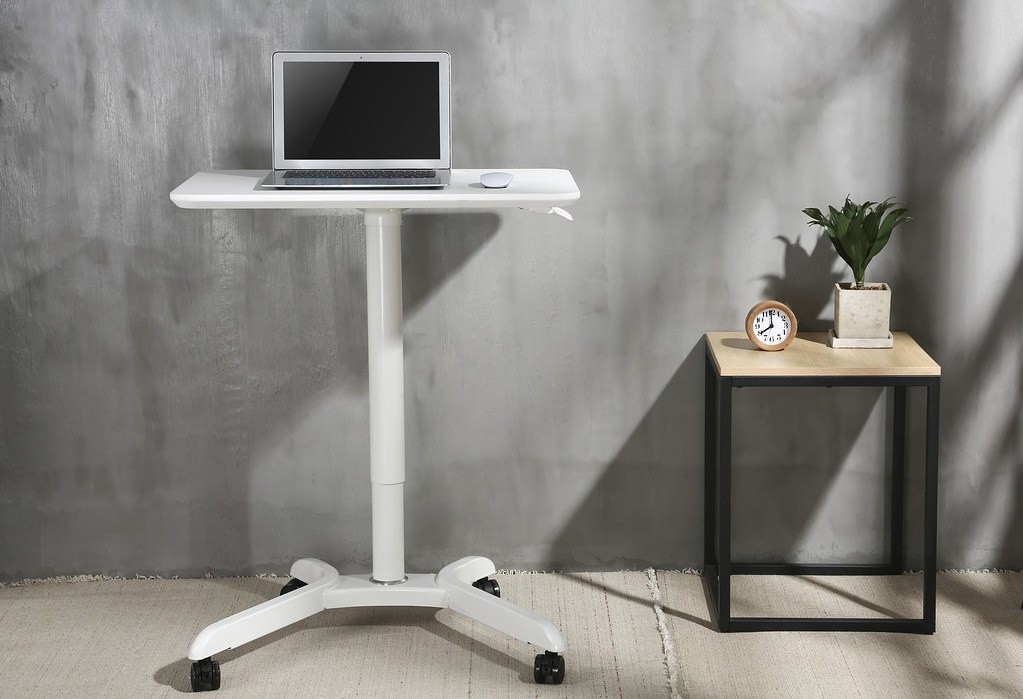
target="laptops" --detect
[261,50,451,187]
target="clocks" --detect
[745,301,797,351]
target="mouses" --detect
[479,172,513,189]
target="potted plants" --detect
[802,192,913,349]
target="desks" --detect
[699,331,941,637]
[168,168,580,692]
